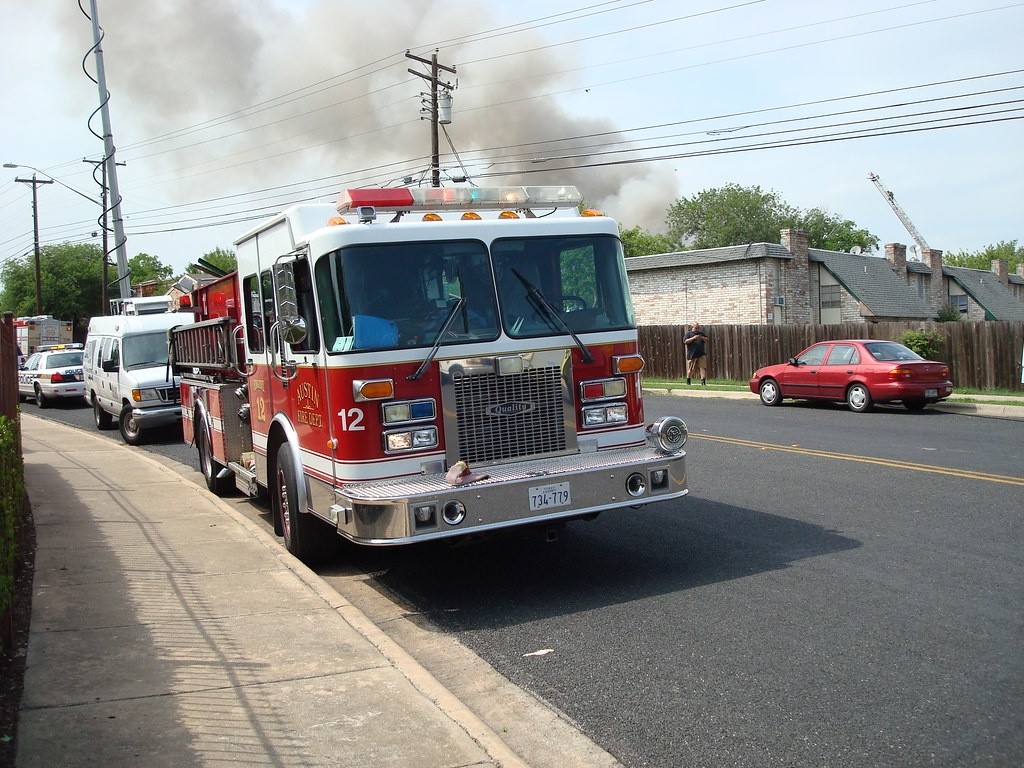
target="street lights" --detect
[0,163,110,320]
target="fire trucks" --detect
[164,179,688,565]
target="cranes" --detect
[866,172,931,253]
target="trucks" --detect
[10,314,74,360]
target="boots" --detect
[687,378,691,385]
[701,379,705,386]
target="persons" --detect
[683,321,709,385]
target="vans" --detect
[82,295,201,444]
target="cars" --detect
[749,339,955,413]
[17,343,91,408]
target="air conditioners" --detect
[774,297,785,306]
